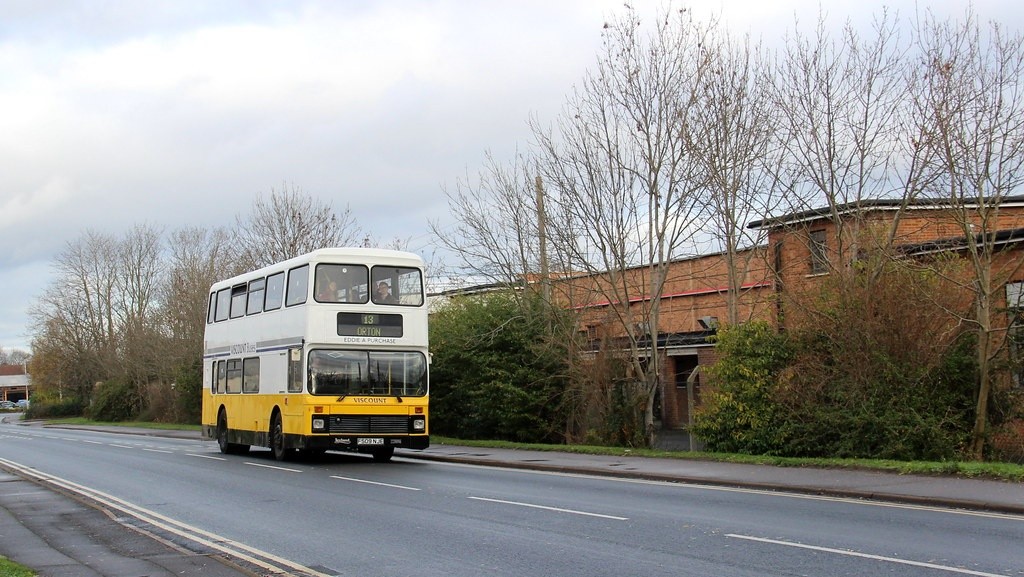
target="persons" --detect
[350,289,360,302]
[362,277,398,305]
[319,281,339,301]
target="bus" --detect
[201,247,433,461]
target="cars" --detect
[15,400,27,407]
[0,401,13,408]
